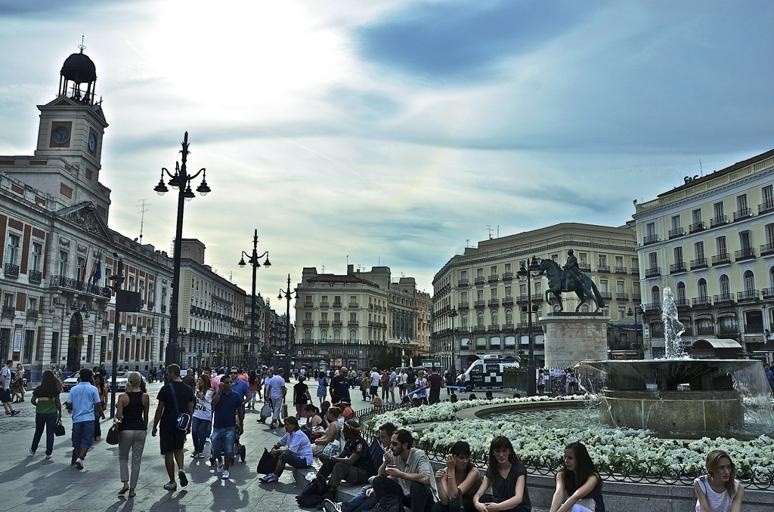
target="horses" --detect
[538,259,605,313]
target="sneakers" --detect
[11,411,20,416]
[76,457,83,469]
[119,485,136,497]
[221,470,229,479]
[179,470,188,487]
[259,475,278,483]
[323,499,342,512]
[164,481,177,490]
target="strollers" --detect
[209,424,246,467]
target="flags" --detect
[91,261,103,283]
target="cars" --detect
[364,359,441,387]
[177,368,217,382]
[105,371,148,392]
[62,370,107,393]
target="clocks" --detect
[89,133,97,152]
[52,126,71,144]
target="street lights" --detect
[765,328,771,366]
[238,229,273,369]
[446,304,460,370]
[178,325,187,367]
[48,303,71,370]
[626,305,649,348]
[515,253,545,397]
[153,130,212,381]
[85,309,103,366]
[276,271,301,384]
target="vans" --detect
[456,354,523,389]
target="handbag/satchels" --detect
[257,448,278,474]
[177,413,191,431]
[261,400,271,417]
[282,404,287,418]
[106,424,119,445]
[55,416,65,436]
[323,442,340,457]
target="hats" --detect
[344,420,361,433]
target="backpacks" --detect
[297,477,326,508]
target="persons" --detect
[764,360,774,391]
[445,368,454,395]
[256,364,286,429]
[456,370,465,386]
[373,430,439,512]
[562,248,579,291]
[29,370,64,458]
[471,436,531,512]
[259,417,313,483]
[564,367,575,396]
[211,375,244,479]
[432,441,482,512]
[151,363,194,491]
[538,370,545,395]
[116,372,149,497]
[70,363,109,470]
[149,366,164,382]
[0,360,25,416]
[694,449,745,512]
[183,365,262,458]
[293,366,443,457]
[548,441,606,512]
[316,423,397,512]
